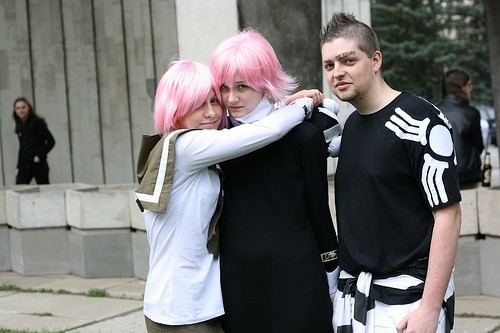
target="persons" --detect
[319,11,462,333]
[209,31,340,333]
[436,69,485,190]
[12,97,56,185]
[134,59,324,333]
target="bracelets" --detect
[289,101,308,121]
